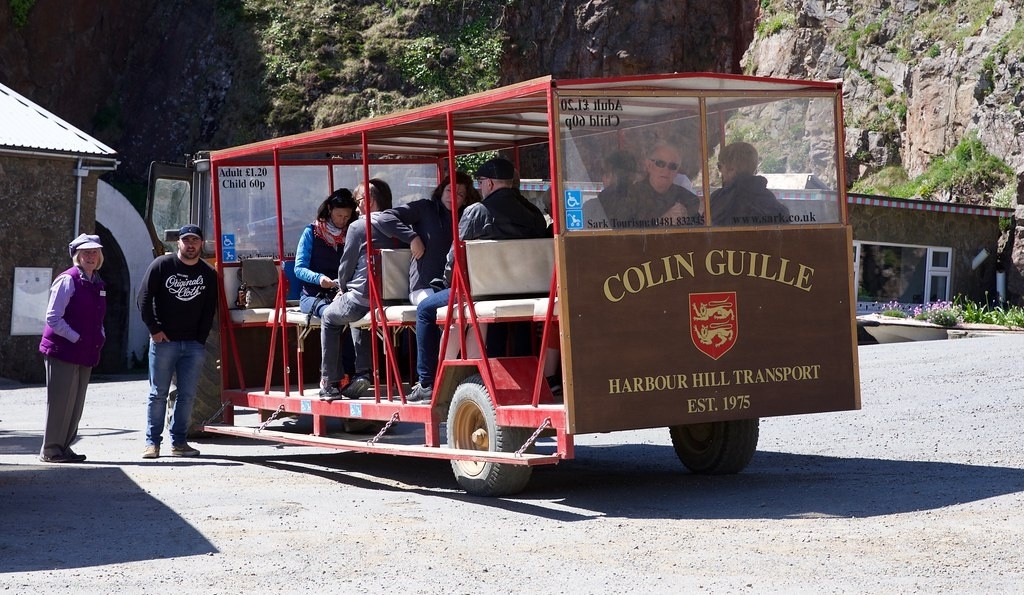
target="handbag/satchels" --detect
[325,287,339,304]
[235,257,279,309]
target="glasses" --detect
[354,198,364,207]
[600,168,606,177]
[717,162,725,172]
[479,178,494,185]
[650,159,678,171]
[544,209,550,214]
[179,226,203,240]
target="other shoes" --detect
[545,375,561,393]
[320,379,326,389]
[41,453,68,463]
[338,373,349,388]
[68,453,86,462]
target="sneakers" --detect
[319,382,342,400]
[340,372,372,399]
[171,443,200,456]
[142,445,160,457]
[406,382,432,404]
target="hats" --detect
[608,150,636,173]
[68,233,103,257]
[473,159,515,179]
[178,224,203,240]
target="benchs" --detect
[229,237,557,400]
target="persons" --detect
[292,158,572,402]
[582,143,790,231]
[38,232,107,464]
[136,223,217,459]
